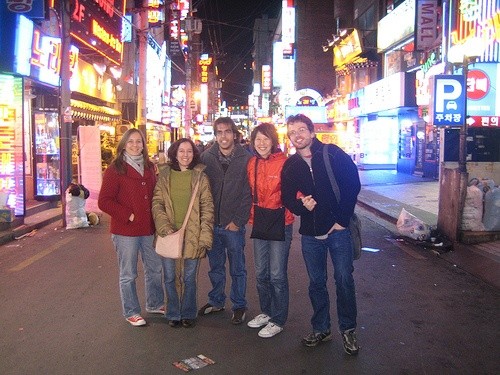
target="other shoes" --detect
[182,320,192,328]
[198,303,225,316]
[169,320,180,328]
[232,310,246,324]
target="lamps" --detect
[321,28,354,53]
[94,60,122,79]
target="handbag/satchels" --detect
[396,208,430,241]
[349,214,363,260]
[250,204,286,242]
[461,178,500,232]
[65,185,90,230]
[155,227,187,258]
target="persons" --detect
[245,123,316,338]
[189,134,280,159]
[150,137,215,328]
[98,127,171,326]
[194,116,249,324]
[281,115,361,356]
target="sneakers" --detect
[247,313,270,328]
[303,329,331,347]
[258,322,283,337]
[148,305,165,314]
[126,314,146,326]
[341,327,359,355]
